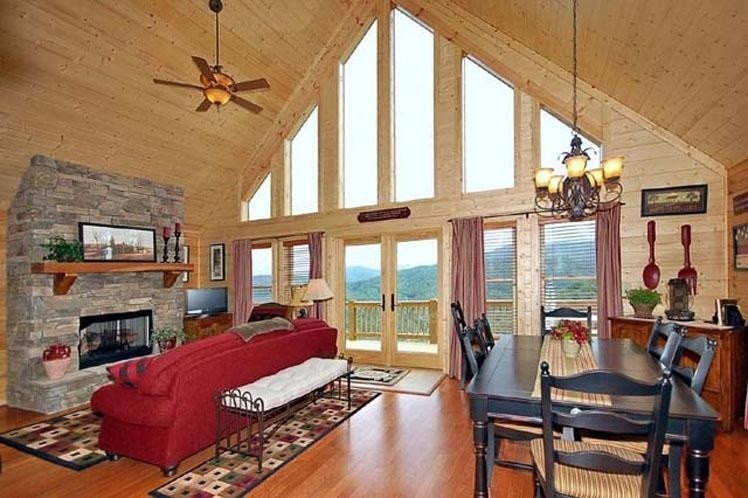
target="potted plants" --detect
[620,288,662,320]
[150,325,190,353]
[33,237,83,264]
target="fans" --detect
[151,0,272,114]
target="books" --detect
[722,304,740,324]
[715,298,736,326]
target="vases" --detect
[559,337,580,358]
[41,358,70,379]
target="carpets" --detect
[1,405,106,472]
[335,362,447,396]
[147,388,381,497]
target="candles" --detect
[164,226,171,238]
[174,222,181,233]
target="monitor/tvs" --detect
[184,287,228,314]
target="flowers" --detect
[42,345,71,361]
[546,317,591,343]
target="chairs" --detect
[461,327,563,497]
[528,360,671,498]
[449,300,474,389]
[579,332,717,497]
[473,313,495,358]
[539,302,593,336]
[645,315,689,372]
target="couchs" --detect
[89,317,338,476]
[246,302,288,324]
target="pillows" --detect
[105,357,157,387]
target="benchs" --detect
[215,354,353,475]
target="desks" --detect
[464,333,722,497]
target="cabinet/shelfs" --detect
[608,313,743,434]
[185,312,234,344]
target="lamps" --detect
[300,278,335,319]
[202,84,231,112]
[533,0,628,224]
[287,282,314,318]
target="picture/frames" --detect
[209,243,226,281]
[640,183,709,218]
[182,244,190,282]
[731,224,748,271]
[78,222,157,262]
